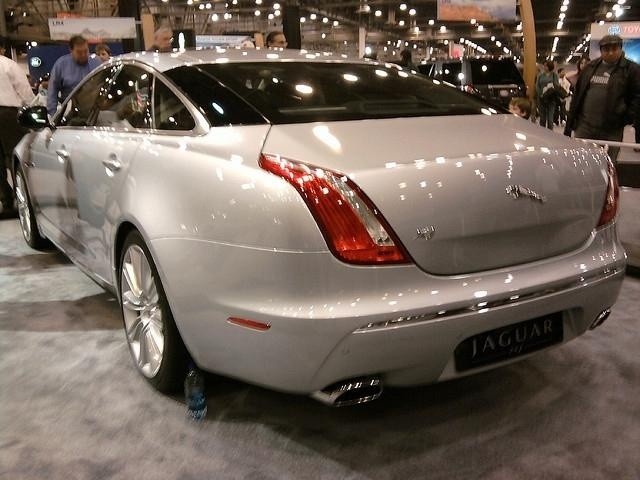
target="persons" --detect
[148,25,174,52]
[508,34,640,161]
[266,30,288,48]
[0,34,113,218]
[399,49,420,73]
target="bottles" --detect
[183,363,208,419]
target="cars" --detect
[9,46,629,412]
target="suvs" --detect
[416,54,531,120]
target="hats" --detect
[599,34,623,47]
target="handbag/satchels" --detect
[553,86,569,99]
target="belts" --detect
[0,105,18,110]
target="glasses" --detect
[273,39,289,44]
